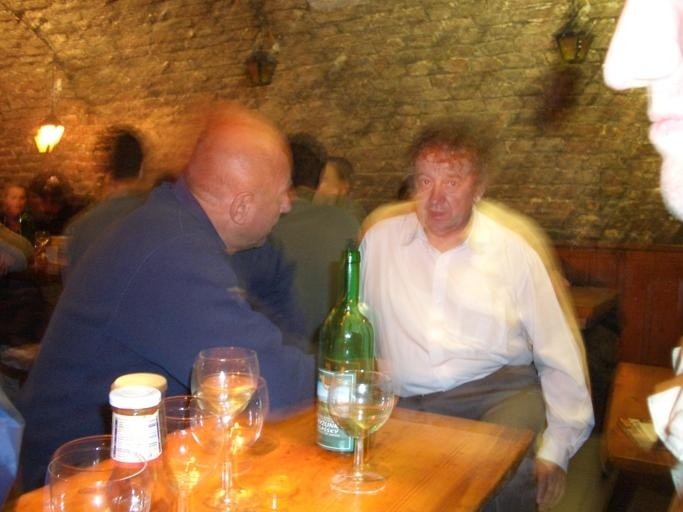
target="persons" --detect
[231,134,358,334]
[599,0,683,511]
[18,101,323,492]
[0,180,28,232]
[62,125,158,287]
[0,213,33,276]
[319,157,368,228]
[29,171,97,238]
[344,117,595,512]
[0,382,25,511]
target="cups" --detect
[327,369,396,495]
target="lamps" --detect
[35,114,66,155]
[552,14,597,63]
[244,46,279,85]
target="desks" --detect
[566,285,620,330]
[598,362,683,511]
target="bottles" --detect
[313,248,374,456]
[20,203,35,247]
[106,384,179,512]
[112,372,175,481]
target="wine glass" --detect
[40,436,153,512]
[158,396,216,511]
[194,347,263,462]
[35,230,50,254]
[201,373,272,510]
